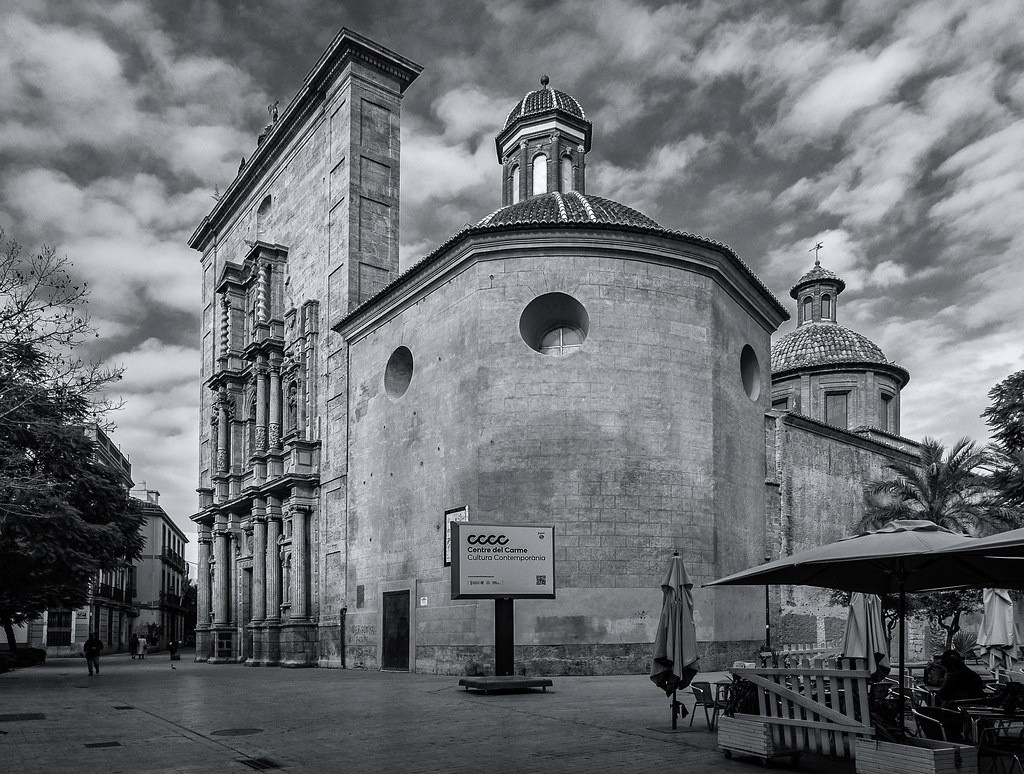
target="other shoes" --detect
[132,657,135,659]
[96,671,99,674]
[88,674,93,676]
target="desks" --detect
[709,682,733,728]
[925,685,941,707]
[958,704,1024,748]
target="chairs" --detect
[690,674,1024,774]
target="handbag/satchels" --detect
[172,652,180,660]
[143,642,148,650]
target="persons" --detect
[128,633,140,659]
[84,633,103,676]
[137,635,147,659]
[934,648,985,708]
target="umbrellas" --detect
[920,525,1024,589]
[974,587,1021,683]
[649,556,702,731]
[839,590,892,693]
[699,517,984,741]
